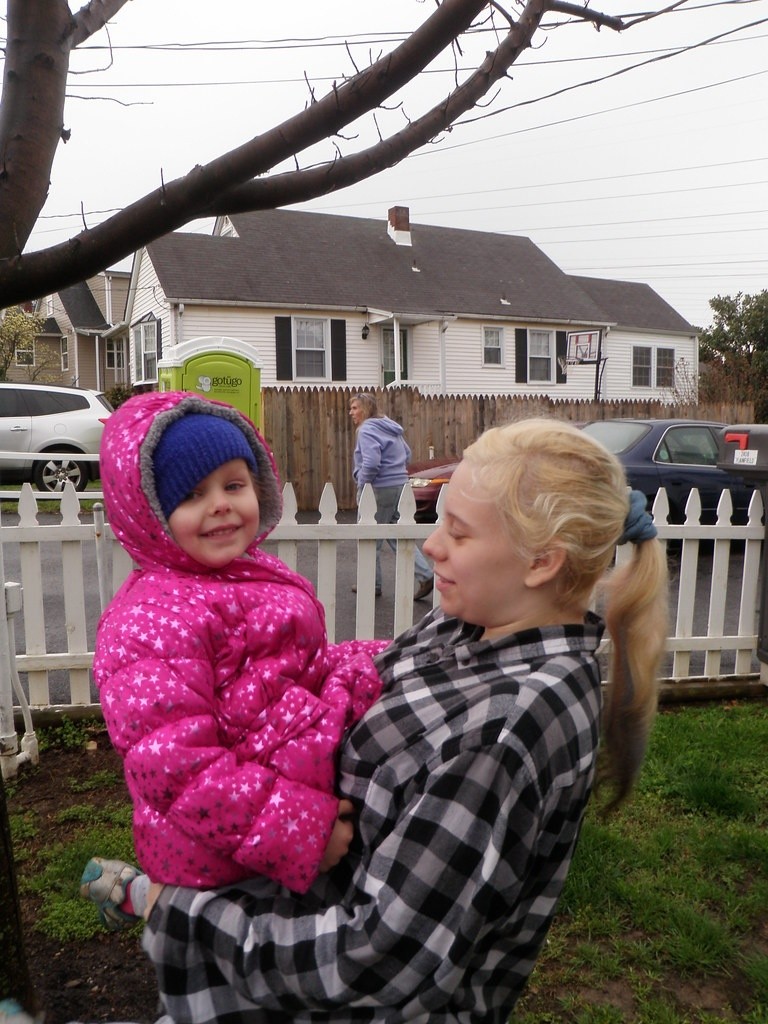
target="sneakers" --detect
[80,857,145,931]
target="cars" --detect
[407,462,461,524]
[576,418,764,552]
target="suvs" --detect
[0,379,115,500]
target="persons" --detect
[349,393,433,600]
[83,417,666,1024]
[94,386,398,895]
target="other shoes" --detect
[351,584,382,596]
[414,576,434,600]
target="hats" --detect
[151,411,259,521]
